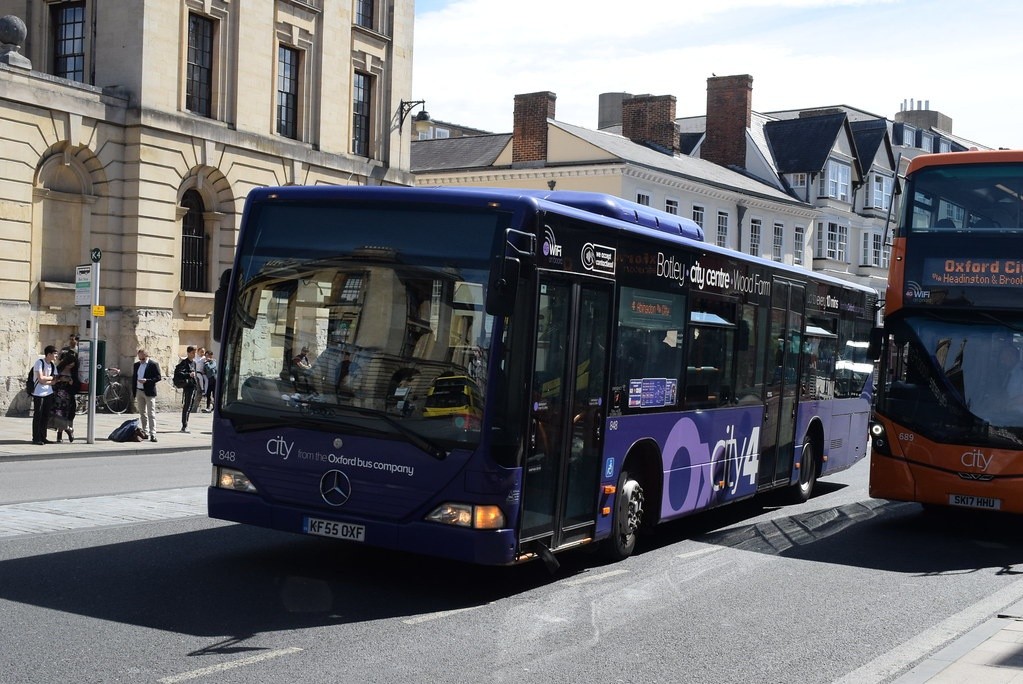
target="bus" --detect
[205,183,880,569]
[866,146,1023,517]
[421,374,485,422]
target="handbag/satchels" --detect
[108,419,145,442]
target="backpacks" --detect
[26,359,54,395]
[173,360,190,388]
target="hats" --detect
[302,346,309,352]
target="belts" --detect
[137,388,144,391]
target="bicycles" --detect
[73,367,130,415]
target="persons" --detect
[31,345,58,444]
[58,332,80,359]
[292,346,314,389]
[132,347,160,442]
[173,344,217,432]
[52,350,78,443]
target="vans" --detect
[833,340,875,393]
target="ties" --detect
[1005,374,1011,390]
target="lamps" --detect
[400,98,435,135]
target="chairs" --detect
[972,217,1001,233]
[934,219,957,233]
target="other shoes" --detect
[33,441,44,445]
[143,435,148,439]
[65,425,74,442]
[43,439,49,443]
[202,407,211,413]
[151,435,158,442]
[57,432,62,442]
[182,427,190,433]
